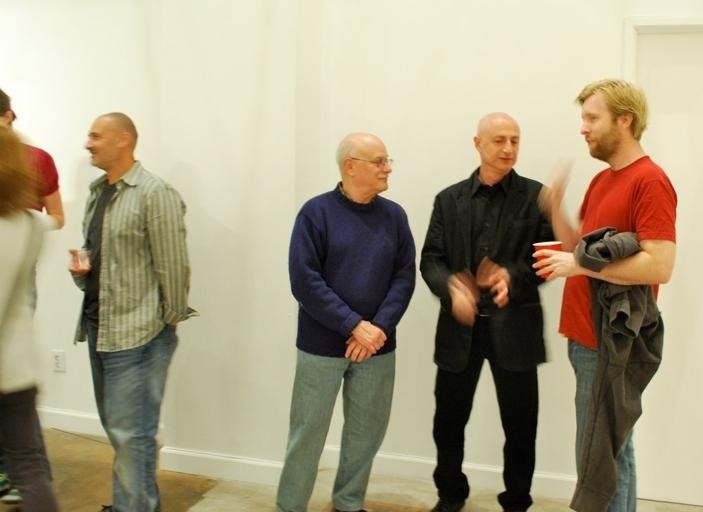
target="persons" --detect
[66,113,199,512]
[0,90,68,504]
[419,112,559,512]
[531,80,677,512]
[2,122,62,512]
[275,131,416,512]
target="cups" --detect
[532,240,563,280]
[67,249,93,272]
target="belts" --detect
[475,306,493,318]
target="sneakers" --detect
[0,471,22,503]
